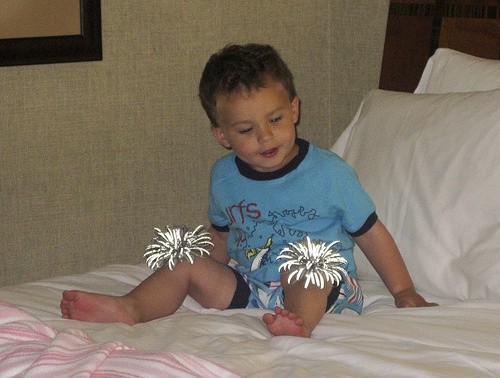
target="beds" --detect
[0,0,500,378]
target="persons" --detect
[61,42,439,338]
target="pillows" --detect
[414,48,500,93]
[329,91,500,300]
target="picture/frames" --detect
[0,0,103,67]
